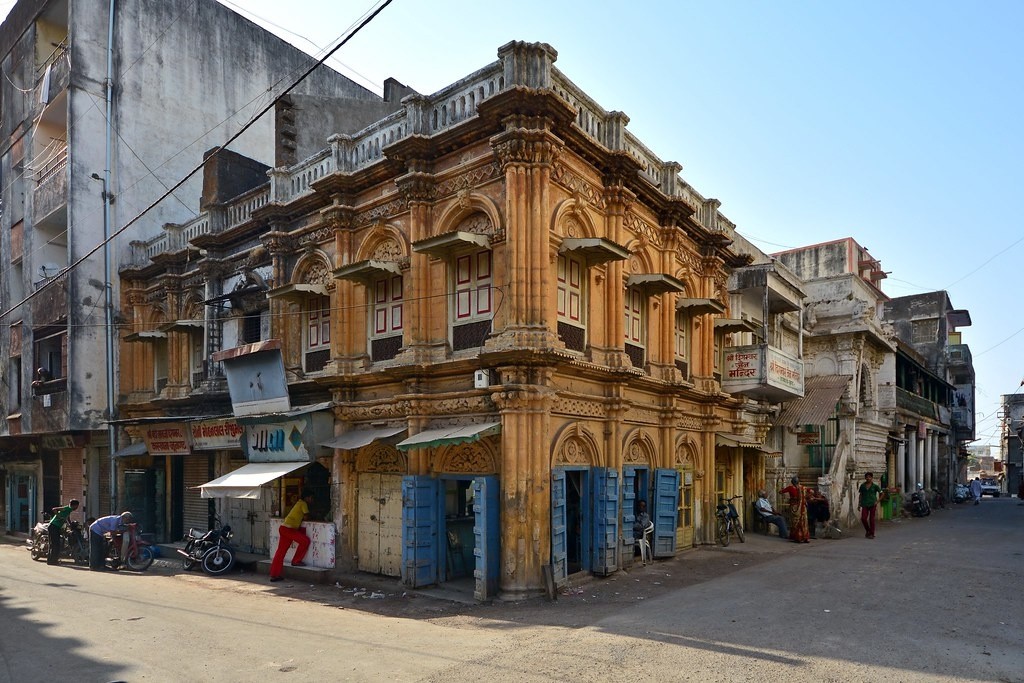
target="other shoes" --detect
[779,535,790,539]
[292,561,306,566]
[865,531,874,539]
[270,576,285,582]
[97,566,113,572]
[805,540,810,543]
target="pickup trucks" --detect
[979,476,1000,498]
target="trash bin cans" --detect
[880,488,901,519]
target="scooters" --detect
[911,483,932,518]
[954,483,970,503]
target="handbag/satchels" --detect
[727,500,740,518]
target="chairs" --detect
[752,501,774,535]
[635,521,655,566]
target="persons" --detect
[269,492,313,582]
[87,517,96,525]
[968,477,996,505]
[89,512,133,571]
[633,500,649,538]
[857,472,884,539]
[47,500,79,565]
[755,490,789,539]
[779,477,814,543]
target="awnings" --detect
[193,285,267,310]
[396,422,501,451]
[320,427,406,450]
[194,462,309,499]
[98,416,210,426]
[755,444,782,457]
[716,434,762,448]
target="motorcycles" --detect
[106,522,154,571]
[176,512,236,575]
[26,512,90,565]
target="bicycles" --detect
[714,495,747,546]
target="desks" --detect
[881,492,901,520]
